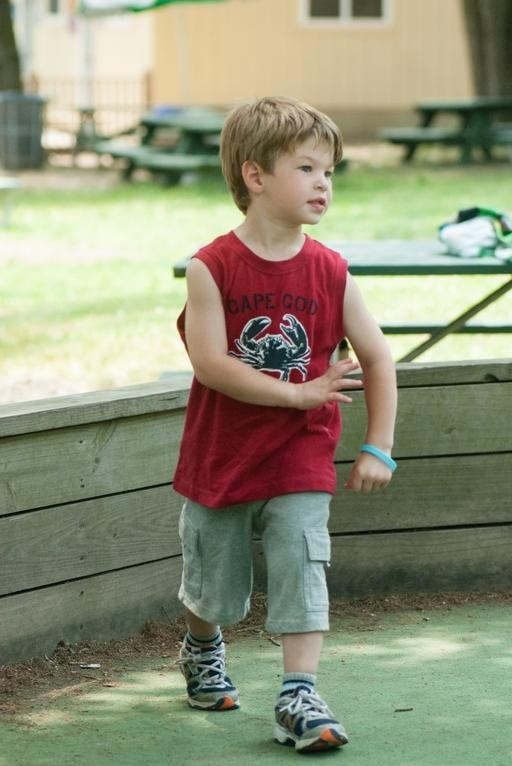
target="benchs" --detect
[382,94,511,160]
[96,112,237,183]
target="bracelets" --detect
[355,442,398,474]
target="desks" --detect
[173,233,512,367]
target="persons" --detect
[161,94,405,758]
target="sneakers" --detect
[270,682,351,755]
[176,633,242,711]
